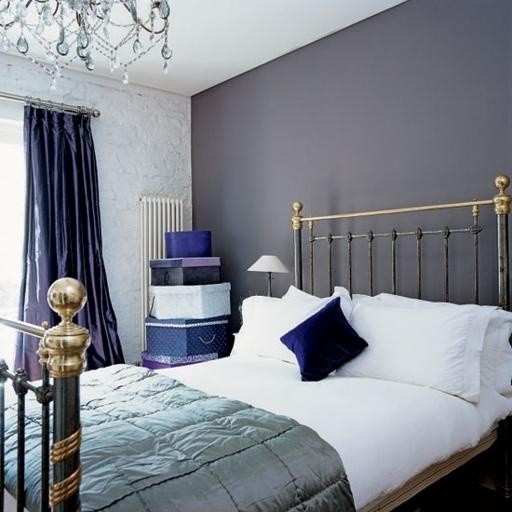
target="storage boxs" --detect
[141,228,232,368]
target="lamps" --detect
[247,253,292,300]
[1,0,172,92]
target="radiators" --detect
[137,193,186,350]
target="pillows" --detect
[235,284,512,409]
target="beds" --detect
[2,177,512,510]
